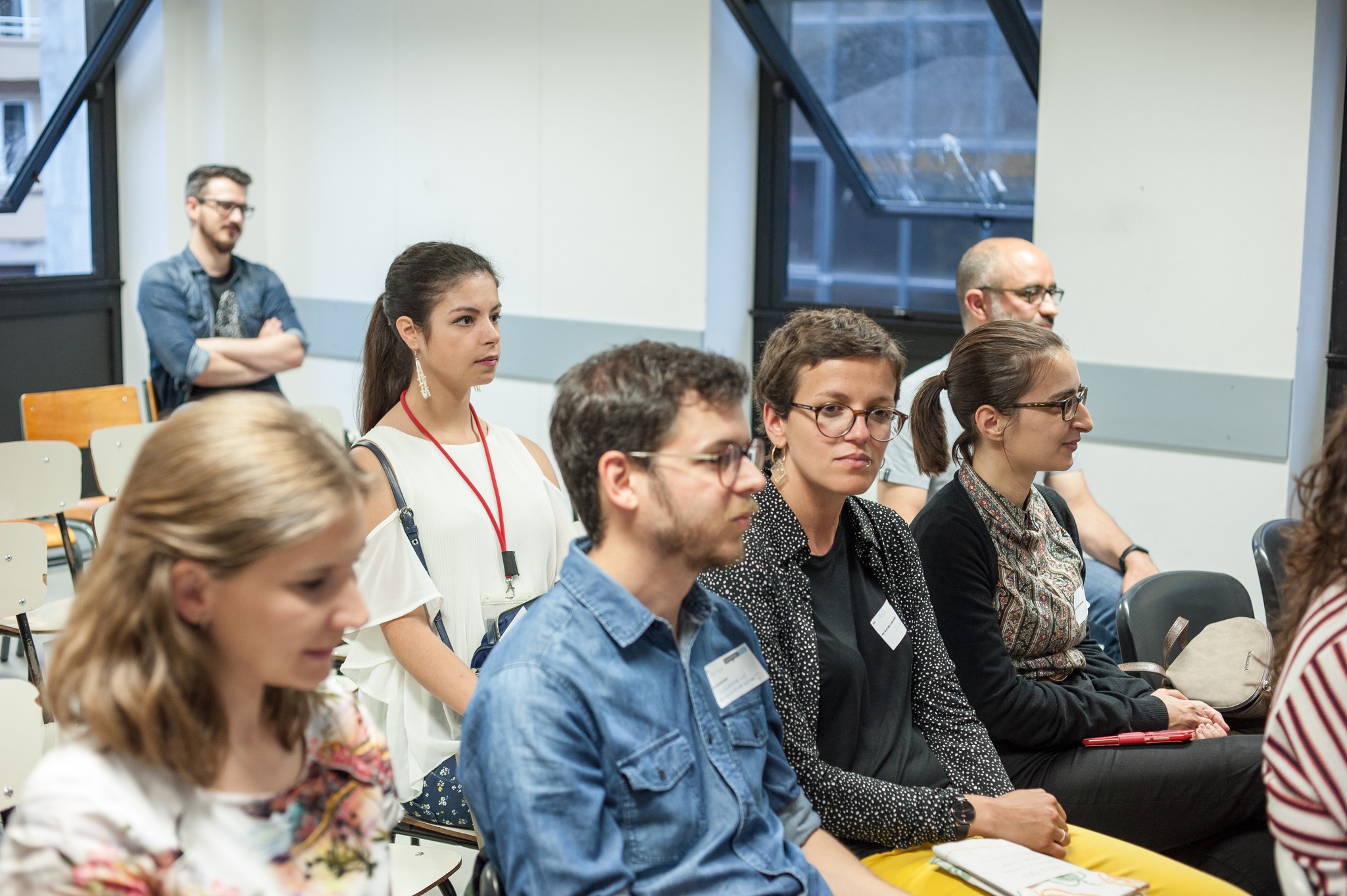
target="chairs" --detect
[0,378,170,815]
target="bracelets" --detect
[1119,544,1149,575]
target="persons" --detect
[341,241,575,896]
[137,163,310,420]
[457,339,903,896]
[1261,400,1347,896]
[880,235,1162,661]
[692,307,1072,862]
[910,319,1281,896]
[2,389,397,896]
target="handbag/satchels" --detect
[471,592,551,671]
[1118,615,1279,733]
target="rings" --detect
[1059,829,1065,843]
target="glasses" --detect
[1008,385,1088,422]
[791,403,909,441]
[626,437,766,488]
[978,285,1064,304]
[198,198,254,219]
